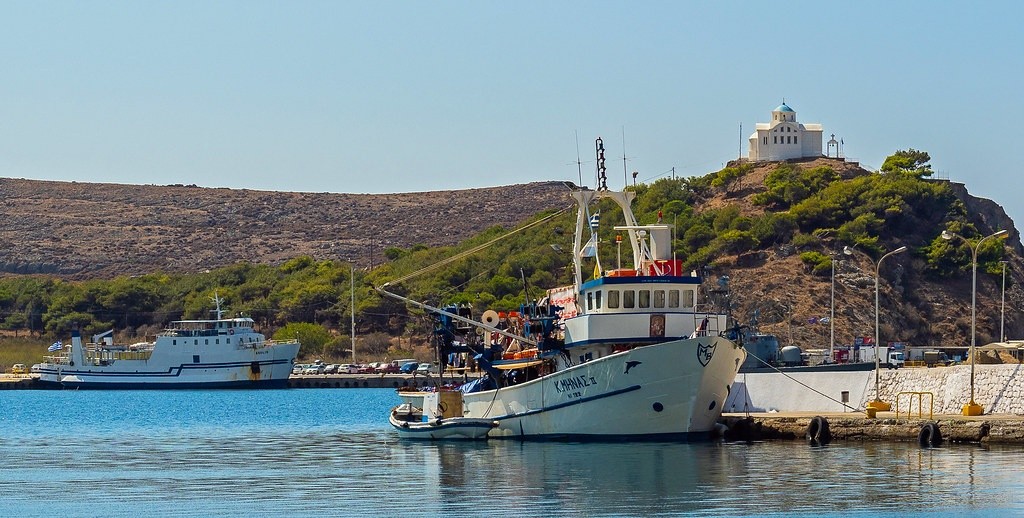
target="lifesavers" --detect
[503,350,534,360]
[70,361,74,366]
[230,329,234,334]
[437,402,448,413]
[806,415,829,443]
[918,423,940,445]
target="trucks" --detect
[835,344,906,370]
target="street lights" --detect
[843,245,909,411]
[941,229,1010,415]
[999,260,1009,342]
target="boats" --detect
[31,292,302,389]
[376,122,780,441]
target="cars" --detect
[292,359,433,374]
[11,364,29,373]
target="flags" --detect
[809,316,829,325]
[582,209,600,257]
[48,340,62,352]
